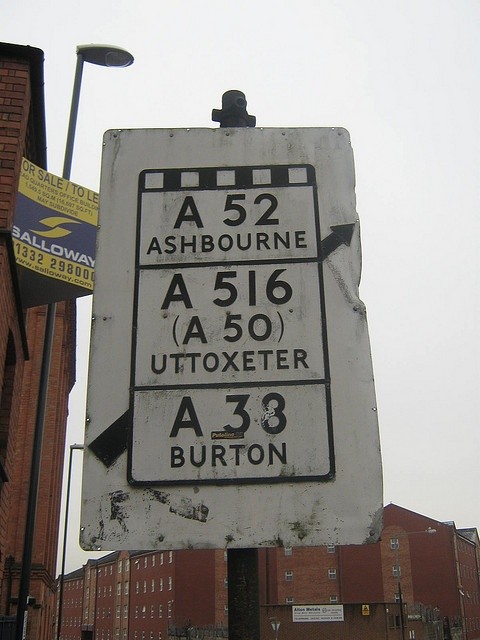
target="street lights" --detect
[14,44,134,640]
[394,528,437,640]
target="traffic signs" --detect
[78,126,383,553]
